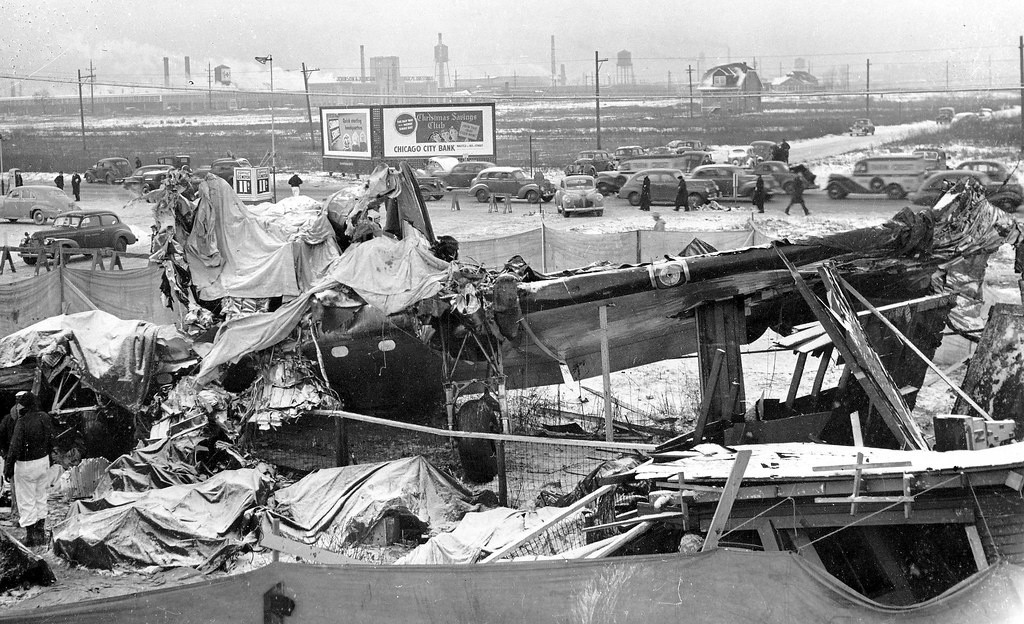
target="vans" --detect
[827,154,925,201]
[595,155,686,195]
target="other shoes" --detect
[785,212,790,215]
[759,211,764,213]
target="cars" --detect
[849,118,875,136]
[913,148,945,171]
[468,167,555,204]
[913,161,1022,214]
[564,150,614,176]
[612,146,648,168]
[688,164,783,201]
[18,209,138,266]
[668,140,777,164]
[936,107,995,126]
[0,186,82,226]
[84,158,133,185]
[430,155,496,191]
[618,169,721,209]
[750,162,817,195]
[125,156,253,186]
[554,175,604,217]
[372,168,449,201]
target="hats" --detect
[15,391,29,403]
[19,392,37,407]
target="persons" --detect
[674,176,689,211]
[288,174,303,196]
[755,174,765,213]
[3,393,51,547]
[785,174,810,215]
[772,139,790,163]
[72,170,81,201]
[639,176,651,211]
[135,157,141,168]
[54,171,64,190]
[702,155,712,165]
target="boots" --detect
[22,523,36,547]
[34,519,46,546]
